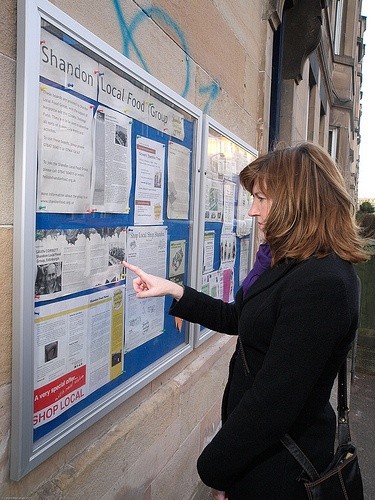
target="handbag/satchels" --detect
[303,442,366,500]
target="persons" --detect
[36,262,63,294]
[122,139,366,500]
